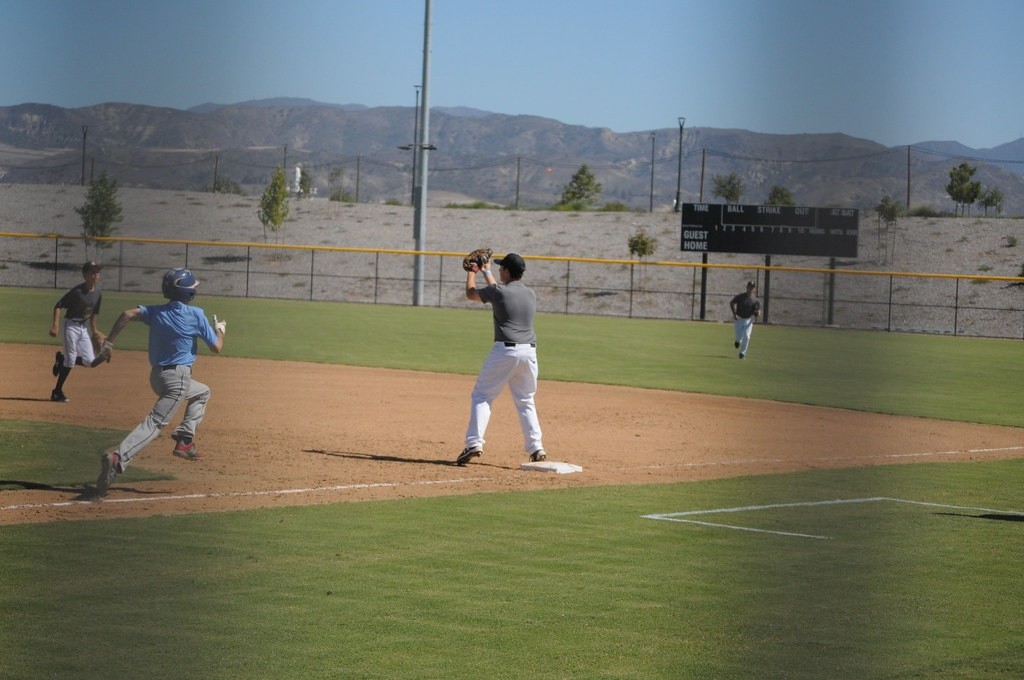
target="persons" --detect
[49,261,106,402]
[730,281,760,359]
[90,268,227,496]
[455,251,547,463]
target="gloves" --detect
[213,315,227,336]
[90,339,113,368]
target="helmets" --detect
[162,266,199,302]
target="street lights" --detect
[649,131,658,213]
[283,142,289,175]
[82,124,89,186]
[674,115,687,212]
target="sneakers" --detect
[53,350,64,376]
[51,389,69,403]
[173,439,206,461]
[97,452,119,495]
[457,446,483,465]
[530,450,546,463]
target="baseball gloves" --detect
[92,333,106,355]
[463,247,493,272]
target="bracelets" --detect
[483,270,491,275]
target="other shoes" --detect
[735,341,740,349]
[740,354,744,360]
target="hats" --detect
[493,253,526,276]
[82,261,104,277]
[748,281,755,287]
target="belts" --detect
[163,365,178,371]
[505,341,535,347]
